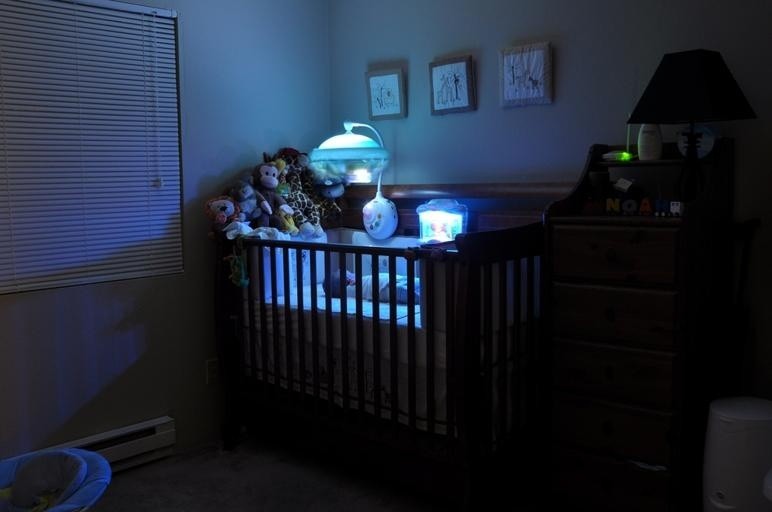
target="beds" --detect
[216,203,547,511]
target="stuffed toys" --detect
[204,148,344,238]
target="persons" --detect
[323,268,420,305]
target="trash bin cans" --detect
[703,397,772,512]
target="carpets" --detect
[98,446,389,511]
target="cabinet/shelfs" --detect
[543,214,733,482]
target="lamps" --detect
[310,121,467,246]
[628,47,759,202]
